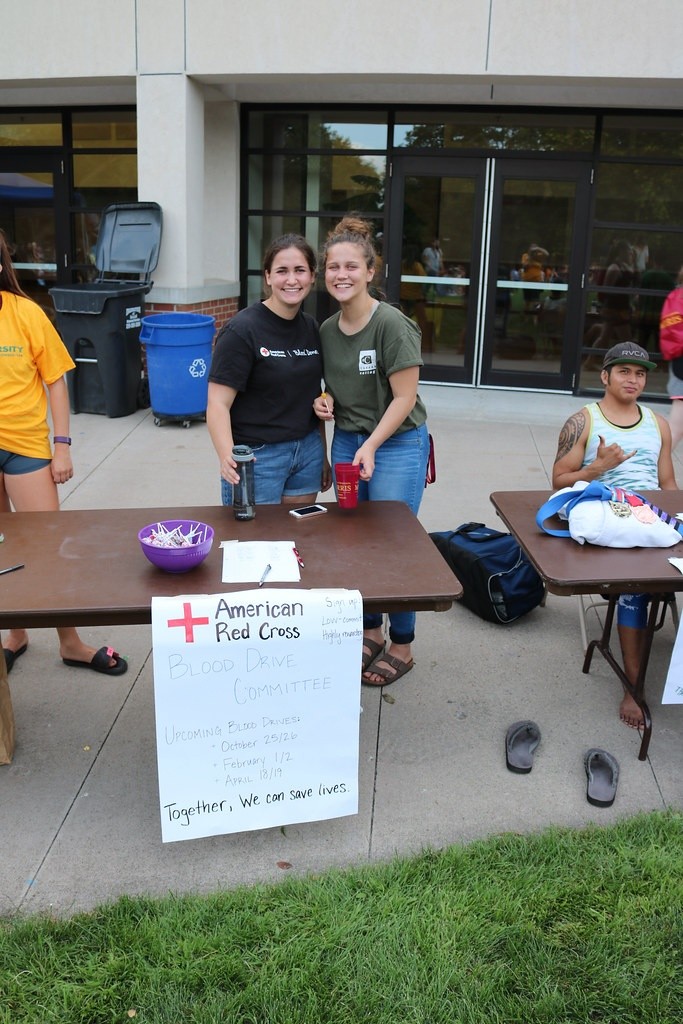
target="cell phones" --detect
[289,504,328,519]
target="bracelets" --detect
[54,436,71,446]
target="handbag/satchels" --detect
[535,480,683,548]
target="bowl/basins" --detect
[137,520,215,574]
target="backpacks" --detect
[425,520,546,624]
[659,286,683,361]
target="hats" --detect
[602,341,657,371]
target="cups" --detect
[334,462,360,510]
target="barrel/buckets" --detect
[139,312,216,422]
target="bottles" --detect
[231,444,256,521]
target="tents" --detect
[0,173,83,209]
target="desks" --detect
[490,490,682,762]
[0,499,463,631]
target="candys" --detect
[142,523,209,548]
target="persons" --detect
[0,229,128,676]
[207,235,332,506]
[659,268,683,453]
[313,212,430,687]
[399,227,446,352]
[521,233,649,370]
[552,342,683,730]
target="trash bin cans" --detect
[48,201,163,419]
[139,313,216,428]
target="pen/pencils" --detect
[292,548,304,568]
[259,564,272,586]
[0,564,24,575]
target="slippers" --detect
[361,654,413,686]
[1,645,28,675]
[62,645,127,677]
[362,637,387,670]
[505,720,541,773]
[584,747,620,807]
